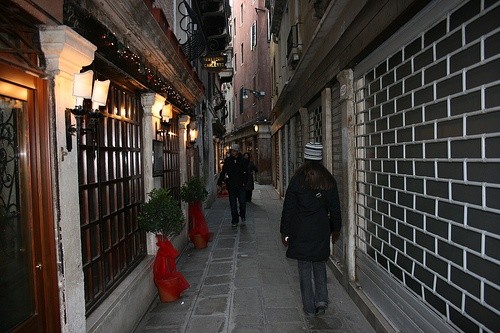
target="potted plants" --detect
[178,176,209,249]
[138,186,189,301]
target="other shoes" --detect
[316,306,326,316]
[304,308,316,317]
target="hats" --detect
[231,144,241,152]
[303,142,324,161]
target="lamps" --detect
[186,129,198,149]
[242,88,265,98]
[155,104,172,138]
[66,71,110,152]
[253,121,272,132]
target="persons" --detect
[215,144,251,228]
[242,152,259,203]
[279,140,342,318]
